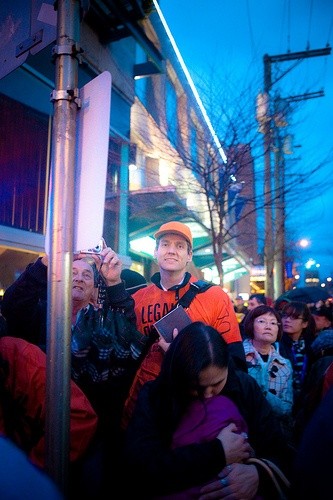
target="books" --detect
[154,305,191,343]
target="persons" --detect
[0,237,333,500]
[130,221,249,373]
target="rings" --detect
[227,465,232,472]
[220,479,226,485]
[242,432,249,442]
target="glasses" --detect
[255,319,281,327]
[281,313,304,320]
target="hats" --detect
[154,221,192,244]
[120,268,148,295]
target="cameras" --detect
[80,240,103,255]
